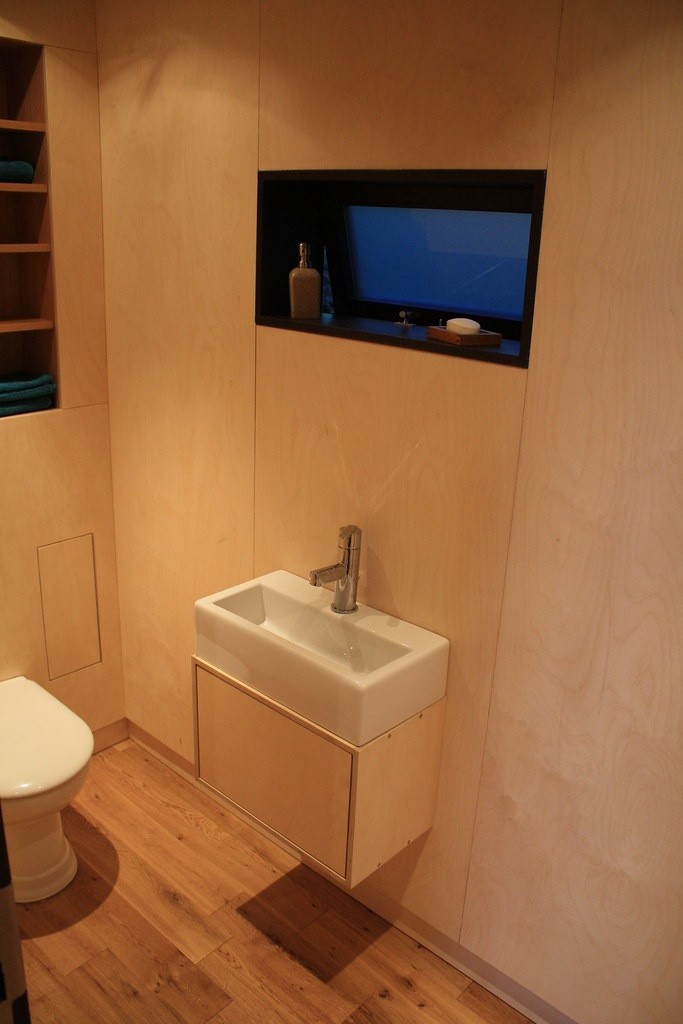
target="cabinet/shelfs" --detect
[0,34,65,421]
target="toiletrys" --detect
[288,242,322,322]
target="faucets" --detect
[308,524,364,615]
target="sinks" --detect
[193,569,450,748]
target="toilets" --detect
[0,675,96,903]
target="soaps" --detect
[446,317,481,336]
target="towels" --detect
[0,373,59,418]
